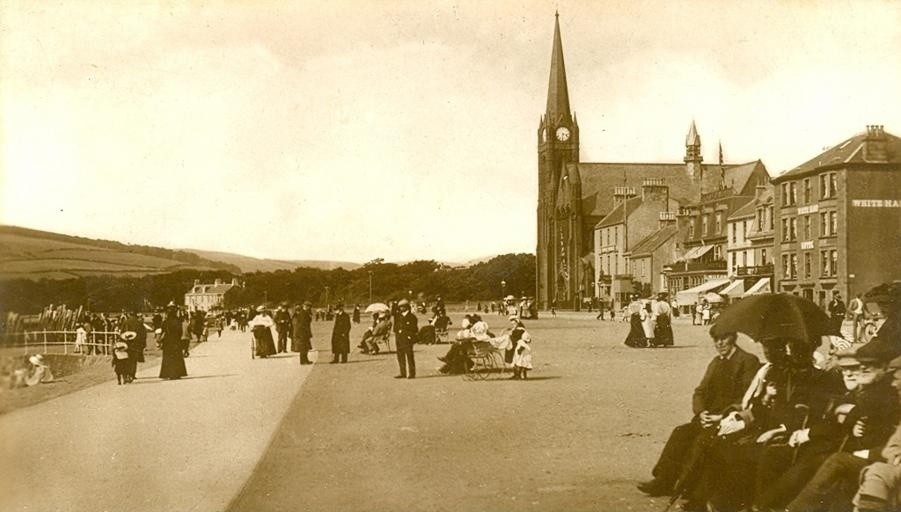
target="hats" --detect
[709,325,736,337]
[836,343,901,369]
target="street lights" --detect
[597,281,603,299]
[590,281,595,298]
[323,286,331,309]
[263,290,269,312]
[366,270,374,303]
[663,266,672,306]
[500,279,508,298]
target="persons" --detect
[26,355,54,386]
[597,296,606,322]
[250,301,314,365]
[330,304,352,363]
[71,302,192,385]
[191,299,256,342]
[358,296,453,353]
[641,279,901,511]
[611,307,616,321]
[479,295,538,319]
[394,300,421,379]
[551,298,557,315]
[624,293,648,349]
[316,303,361,323]
[437,314,533,380]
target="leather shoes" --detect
[637,477,678,495]
[395,375,415,378]
[330,360,347,363]
[300,360,313,364]
